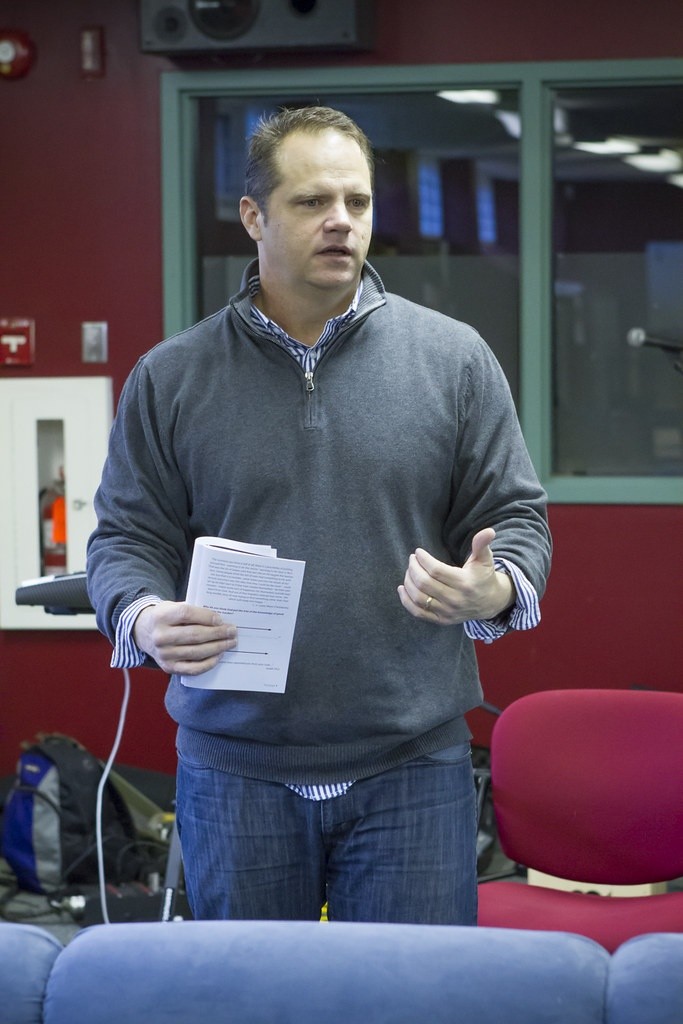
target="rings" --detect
[423,595,433,611]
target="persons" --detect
[84,107,553,929]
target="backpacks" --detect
[1,734,126,893]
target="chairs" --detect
[475,688,683,955]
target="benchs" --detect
[0,918,683,1022]
